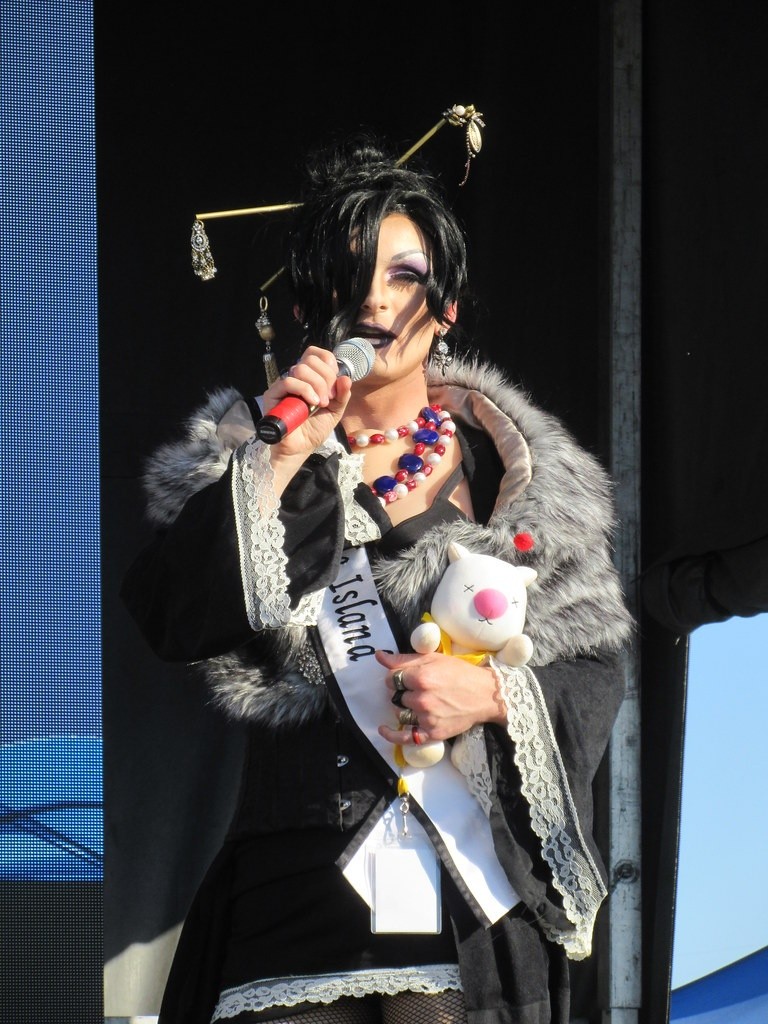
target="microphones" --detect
[257,336,375,445]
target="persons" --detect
[144,138,627,1024]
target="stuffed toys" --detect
[394,541,538,776]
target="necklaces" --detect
[346,403,457,509]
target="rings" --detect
[399,709,412,725]
[392,670,407,692]
[279,370,291,379]
[412,726,420,747]
[391,688,407,709]
